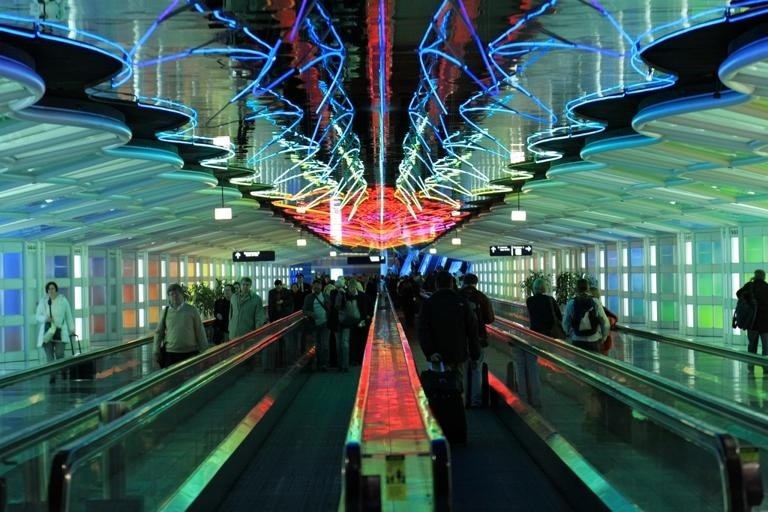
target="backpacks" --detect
[569,295,600,336]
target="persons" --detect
[562,279,610,351]
[385,271,464,329]
[213,277,266,372]
[153,285,210,365]
[737,270,768,379]
[510,342,542,409]
[269,273,379,372]
[37,282,75,384]
[417,269,493,409]
[526,278,563,337]
[589,286,618,355]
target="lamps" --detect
[451,192,526,246]
[215,187,307,247]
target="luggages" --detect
[69,334,93,393]
[421,357,467,445]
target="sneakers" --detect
[316,360,351,374]
[50,369,67,383]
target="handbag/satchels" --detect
[157,345,166,369]
[212,319,225,345]
[338,300,361,327]
[733,282,757,330]
[43,319,61,344]
[551,320,566,339]
